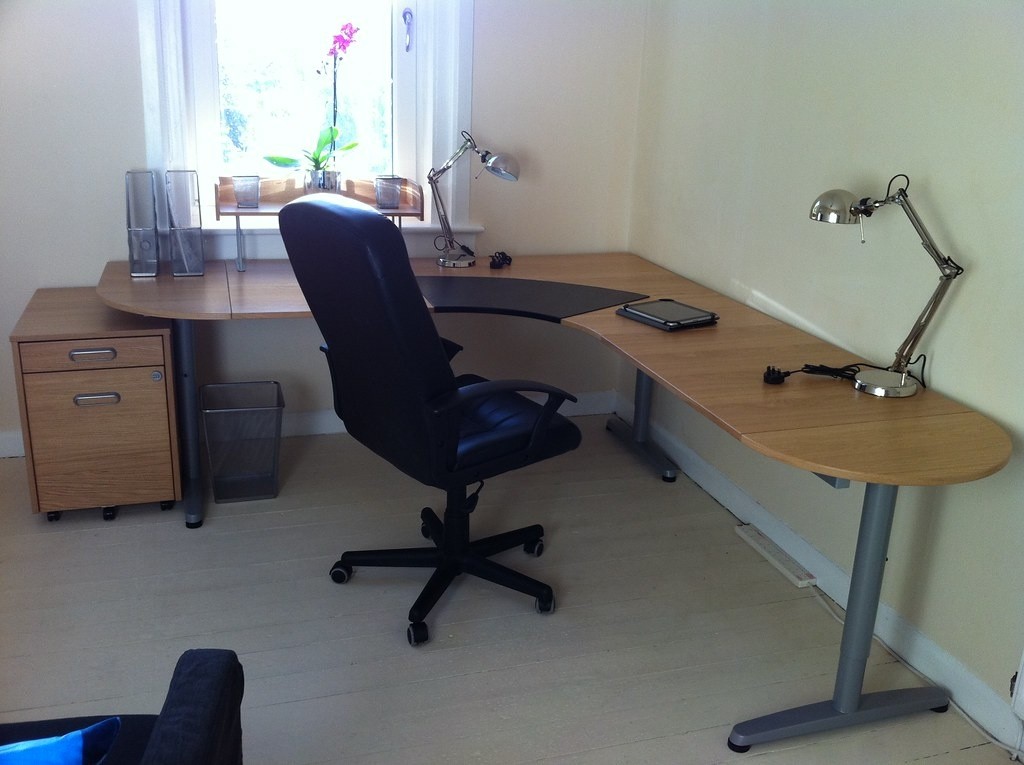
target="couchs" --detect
[0,648,247,765]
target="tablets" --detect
[621,298,720,327]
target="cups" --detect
[232,175,261,209]
[376,175,403,209]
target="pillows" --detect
[0,717,122,765]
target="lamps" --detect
[763,173,965,398]
[426,131,521,269]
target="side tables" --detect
[8,285,183,522]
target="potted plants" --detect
[263,126,360,195]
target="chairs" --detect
[278,192,580,647]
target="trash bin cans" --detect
[199,380,286,503]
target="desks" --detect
[94,251,1014,755]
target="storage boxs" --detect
[375,175,402,209]
[232,175,260,207]
[124,170,160,277]
[164,170,204,276]
